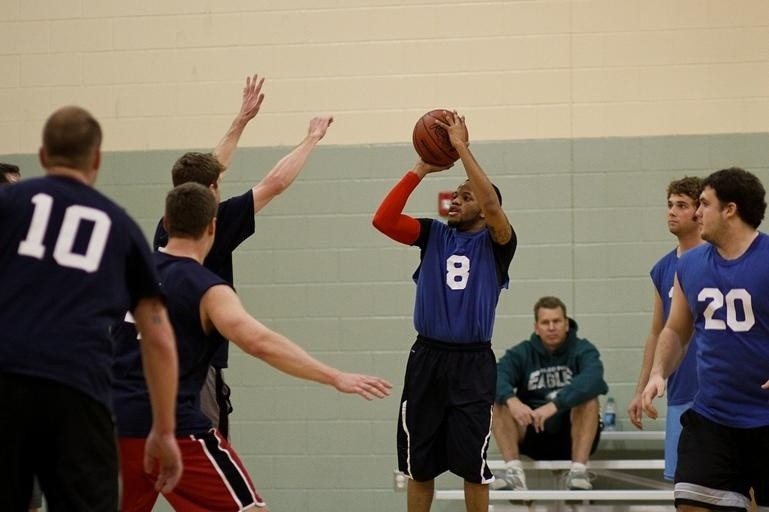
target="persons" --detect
[628,171,706,486]
[0,161,44,512]
[370,106,517,512]
[490,293,611,492]
[105,180,396,512]
[149,69,336,444]
[638,164,769,512]
[1,105,183,512]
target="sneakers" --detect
[567,469,592,490]
[492,469,528,492]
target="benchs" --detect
[433,427,684,512]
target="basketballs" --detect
[412,109,468,165]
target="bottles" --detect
[601,397,618,432]
